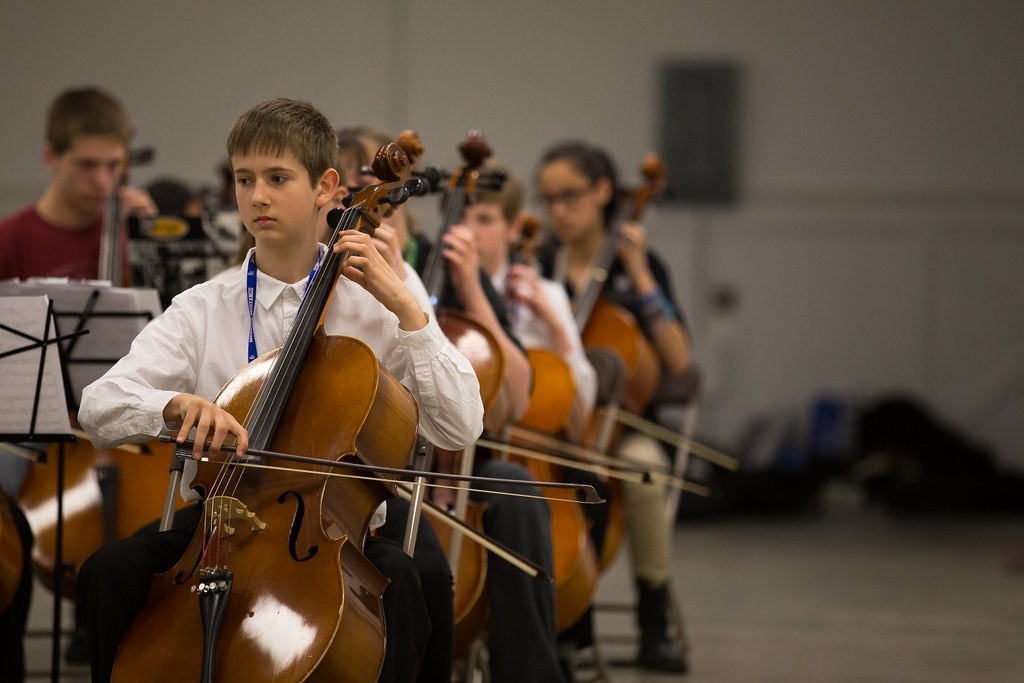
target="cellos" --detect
[0,130,663,683]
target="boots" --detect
[633,575,690,672]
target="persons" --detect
[0,86,703,683]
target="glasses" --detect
[540,184,596,212]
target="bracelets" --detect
[641,289,674,322]
